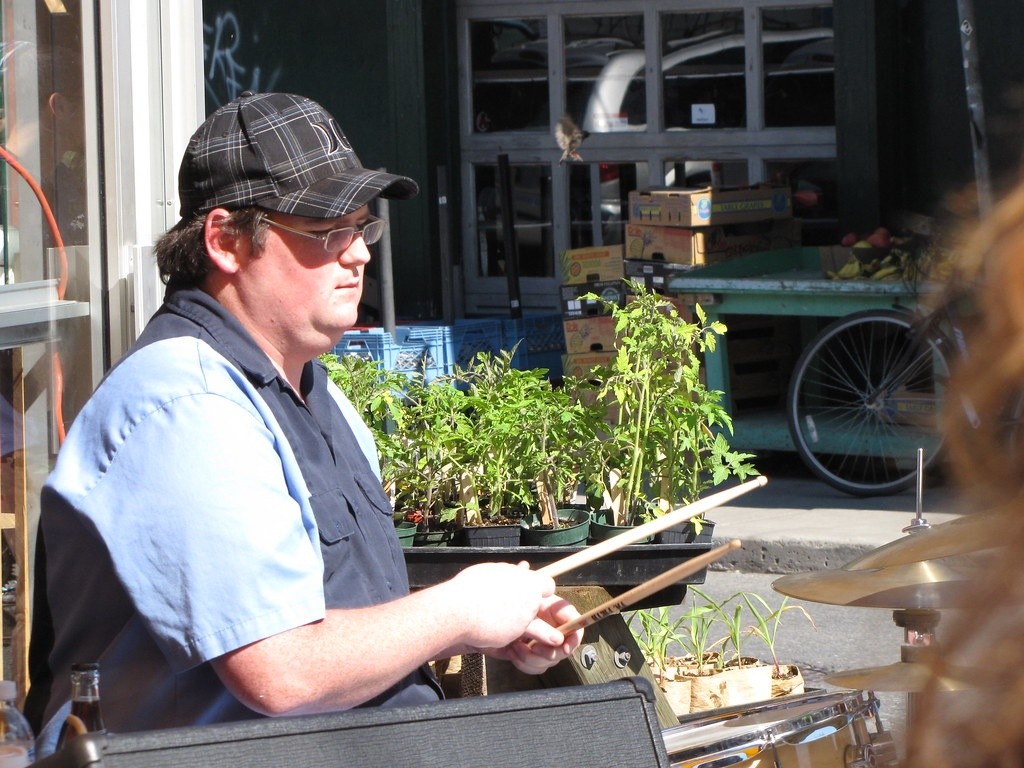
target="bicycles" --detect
[787,235,979,498]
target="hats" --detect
[177,91,419,218]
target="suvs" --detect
[472,18,836,274]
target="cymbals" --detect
[772,557,1005,609]
[822,663,980,694]
[842,502,1024,570]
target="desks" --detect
[667,248,950,458]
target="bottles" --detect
[56,663,105,752]
[0,681,36,768]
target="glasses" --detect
[260,214,387,258]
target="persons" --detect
[24,90,584,738]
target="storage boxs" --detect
[404,543,711,614]
[875,385,937,428]
[326,311,568,408]
[559,185,804,421]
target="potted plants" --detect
[318,279,761,547]
[622,586,819,716]
